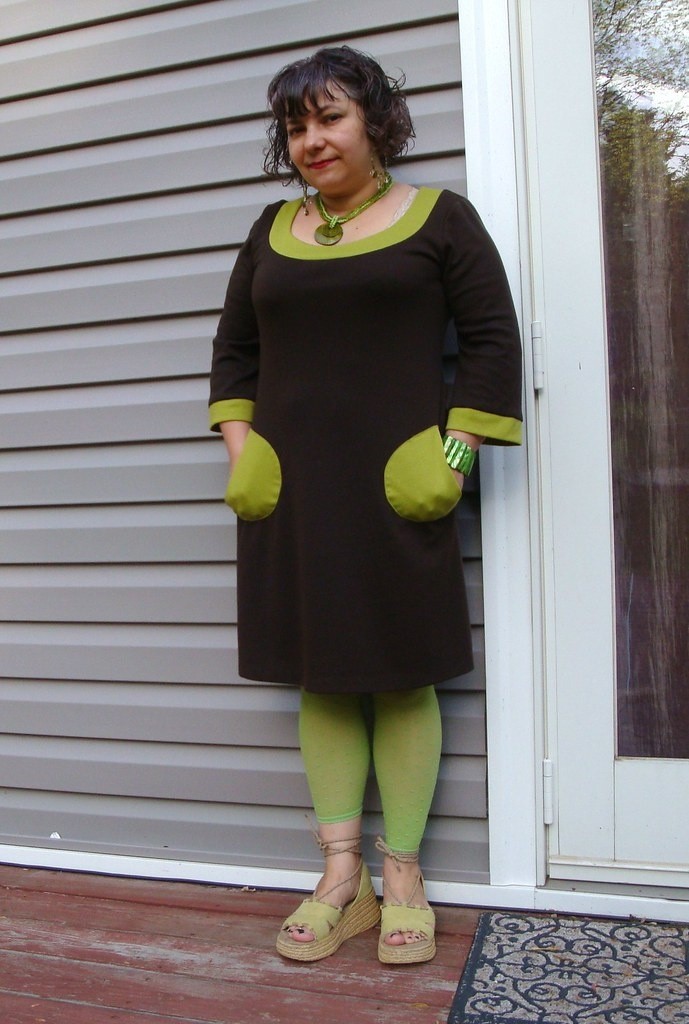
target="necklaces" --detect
[314,172,392,246]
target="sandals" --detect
[276,812,379,962]
[375,836,436,963]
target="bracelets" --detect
[441,433,477,477]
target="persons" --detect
[206,45,522,965]
